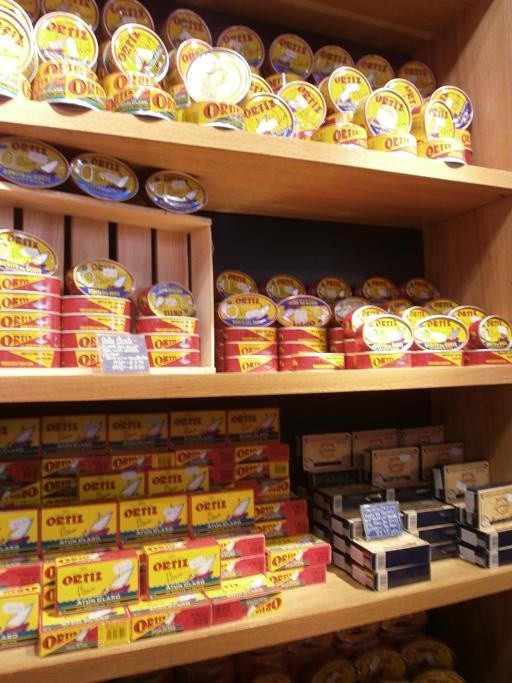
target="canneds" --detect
[0,228,202,368]
[0,137,208,215]
[215,270,512,372]
[0,0,475,165]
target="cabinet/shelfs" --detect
[0,0,512,683]
